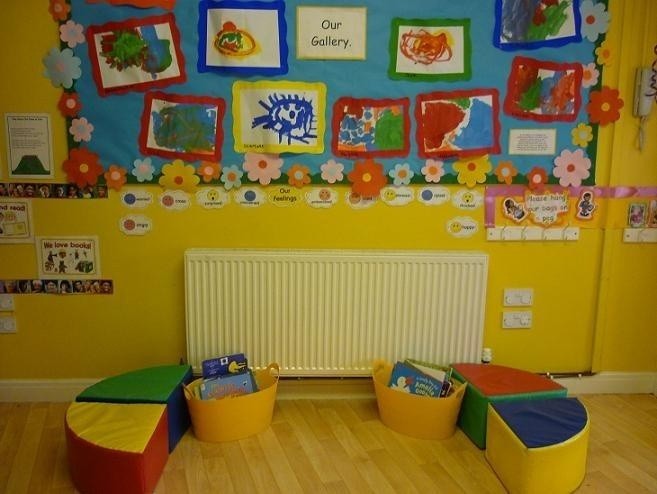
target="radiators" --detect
[183,252,495,380]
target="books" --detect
[194,353,257,399]
[387,359,453,398]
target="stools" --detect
[449,360,593,493]
[63,365,194,494]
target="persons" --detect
[0,279,113,294]
[0,183,108,198]
